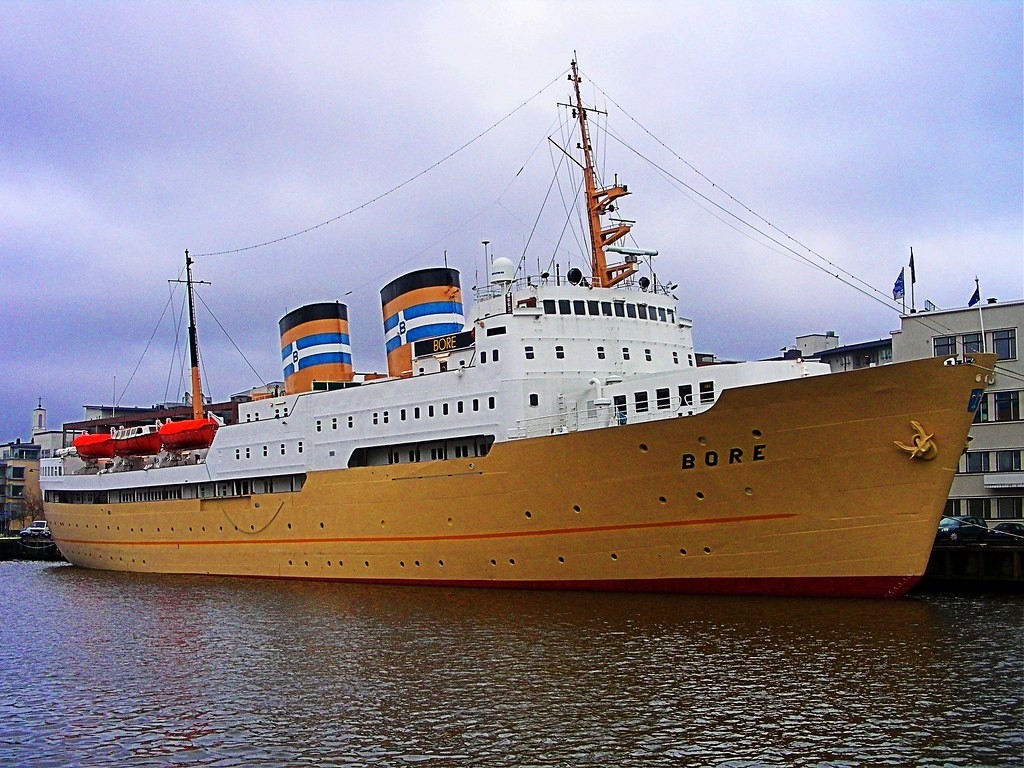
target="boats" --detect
[38,45,998,599]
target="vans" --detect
[31,521,51,536]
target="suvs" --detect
[936,515,988,543]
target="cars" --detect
[20,527,32,538]
[987,522,1024,545]
